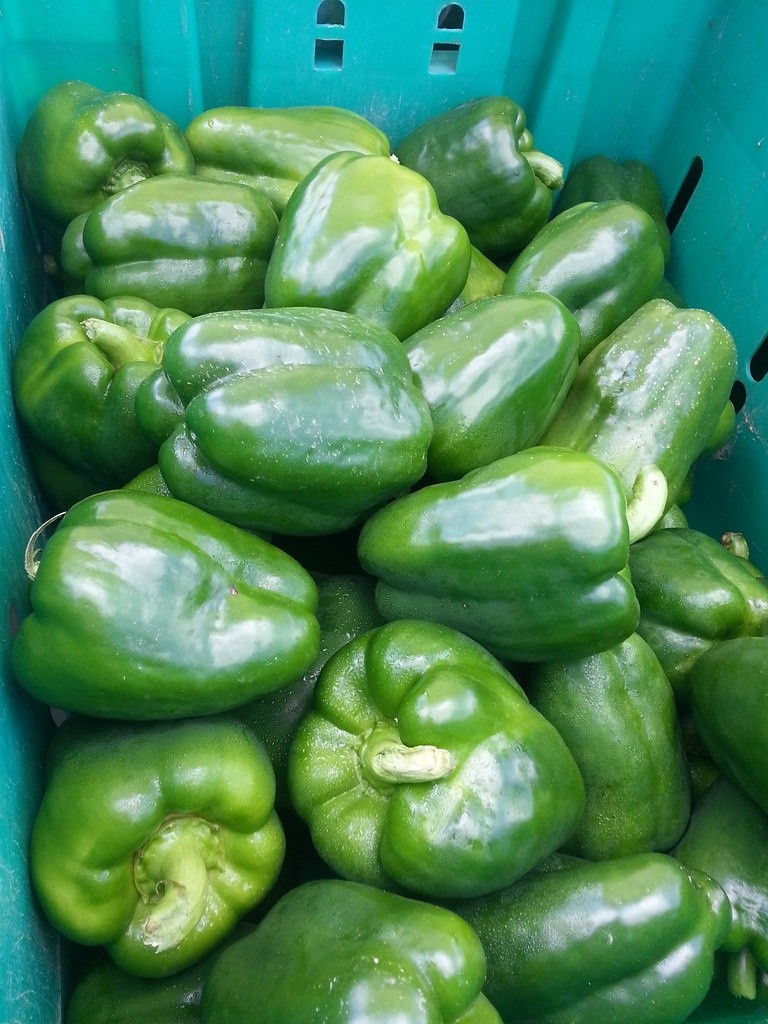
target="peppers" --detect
[12,80,766,1022]
